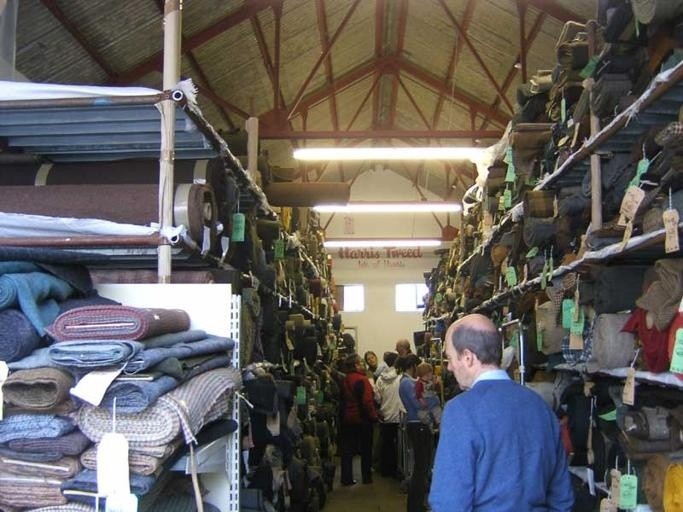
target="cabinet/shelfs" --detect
[1,0,345,512]
[417,20,683,512]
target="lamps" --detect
[291,147,482,160]
[323,239,442,249]
[311,200,463,214]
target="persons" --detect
[323,333,442,511]
[427,313,576,512]
